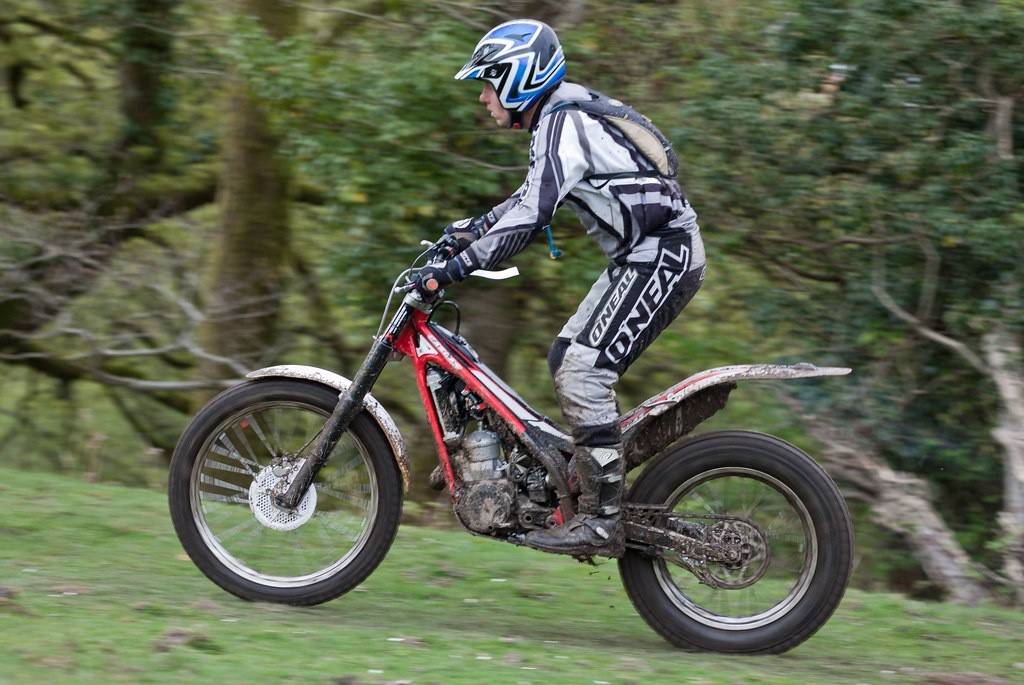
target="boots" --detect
[526,439,626,558]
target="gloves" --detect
[443,210,498,250]
[415,247,482,303]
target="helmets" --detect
[454,19,566,129]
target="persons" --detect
[414,19,707,557]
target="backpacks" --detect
[543,86,681,189]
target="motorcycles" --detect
[165,237,857,658]
